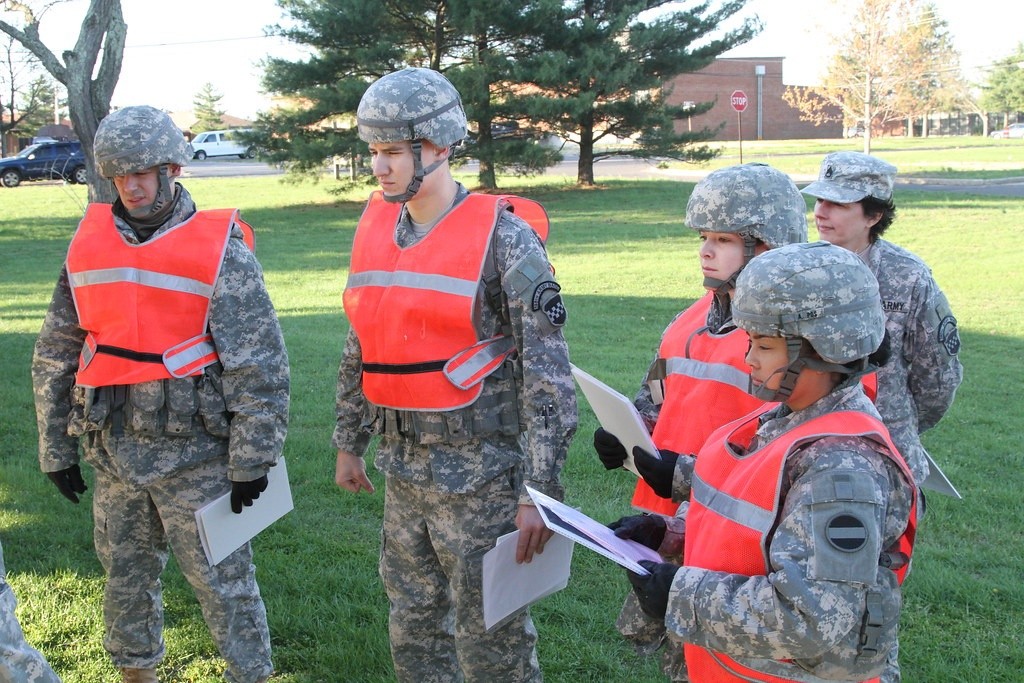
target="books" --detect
[571,363,662,478]
[524,485,662,576]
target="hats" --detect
[801,150,898,205]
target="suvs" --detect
[0,136,88,188]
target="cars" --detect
[990,122,1024,139]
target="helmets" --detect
[730,242,887,365]
[684,163,808,251]
[356,67,468,149]
[94,105,194,179]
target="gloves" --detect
[593,426,628,472]
[626,559,680,623]
[46,464,89,504]
[230,475,269,514]
[633,446,679,499]
[606,515,667,552]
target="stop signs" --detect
[730,90,748,112]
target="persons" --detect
[333,68,578,682]
[31,104,290,683]
[801,150,963,586]
[593,167,930,683]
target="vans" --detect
[189,129,259,161]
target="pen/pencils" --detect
[542,404,547,429]
[549,405,552,416]
